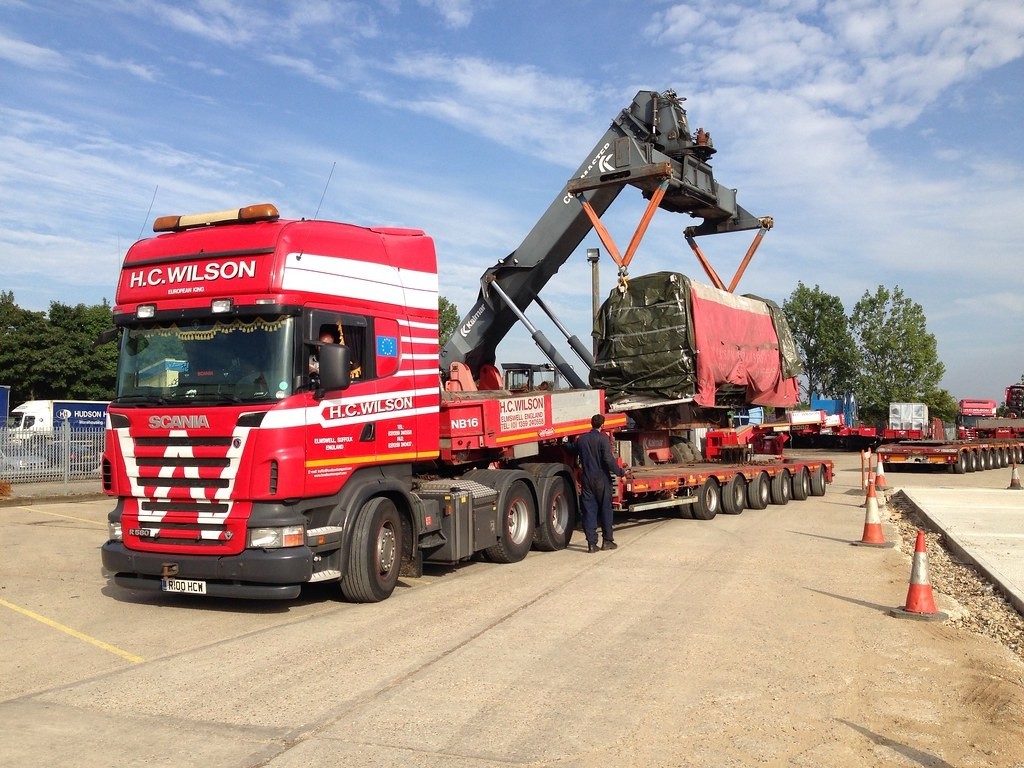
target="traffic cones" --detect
[1007,461,1024,490]
[889,530,950,621]
[872,453,894,492]
[850,479,897,549]
[858,470,885,508]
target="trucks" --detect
[7,399,113,441]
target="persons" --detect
[537,381,549,390]
[308,332,334,374]
[521,379,534,390]
[563,414,629,553]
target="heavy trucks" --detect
[876,385,1024,475]
[92,84,838,607]
[743,382,885,456]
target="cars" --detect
[0,445,50,472]
[38,445,101,472]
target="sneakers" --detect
[588,542,599,552]
[601,539,617,549]
